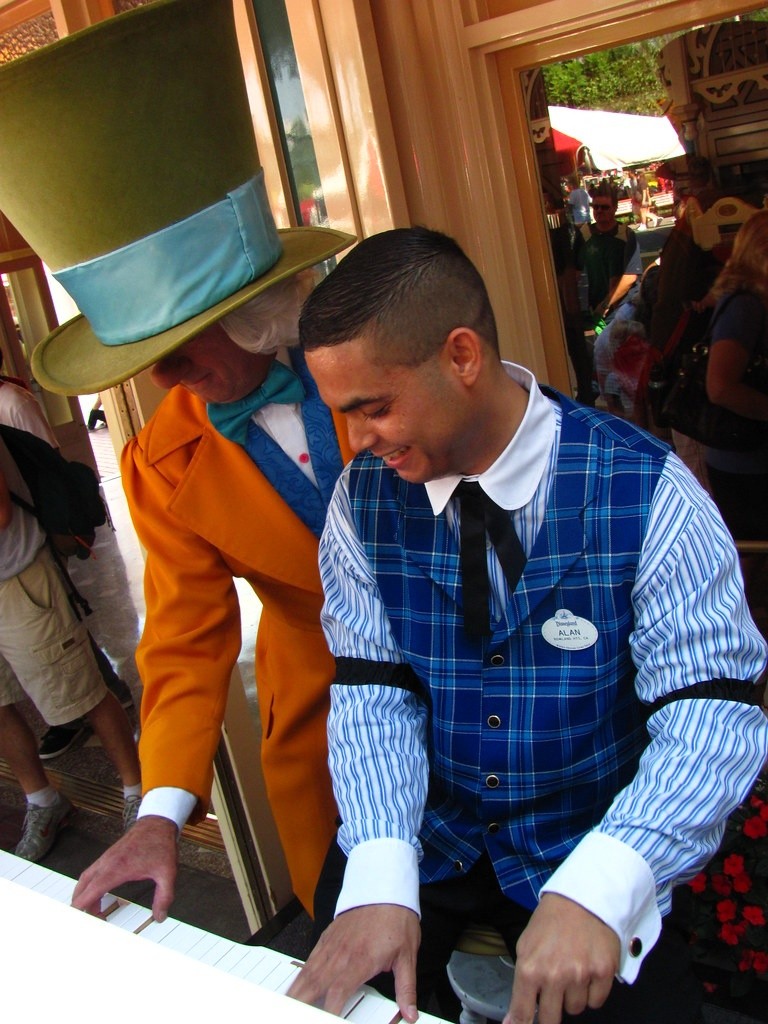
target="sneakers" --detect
[15,792,77,862]
[123,795,142,833]
[107,678,133,709]
[38,719,84,759]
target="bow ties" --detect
[449,479,528,653]
[206,359,306,446]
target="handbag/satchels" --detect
[660,288,768,453]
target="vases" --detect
[699,968,768,1024]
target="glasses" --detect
[592,204,613,211]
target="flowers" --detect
[686,780,768,996]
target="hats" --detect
[0,0,357,396]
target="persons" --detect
[0,430,143,861]
[84,393,108,434]
[1,350,134,760]
[559,168,768,619]
[32,196,347,963]
[282,224,768,1024]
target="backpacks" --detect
[0,418,116,617]
